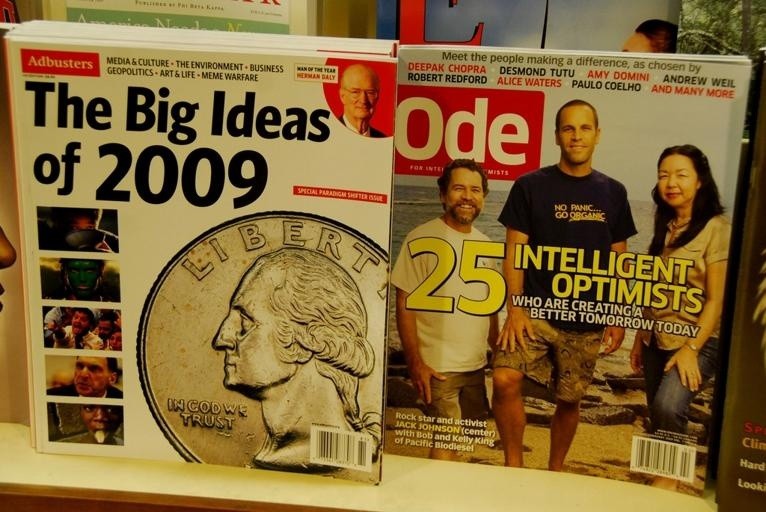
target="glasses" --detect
[343,86,379,100]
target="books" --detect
[0,0,766,511]
